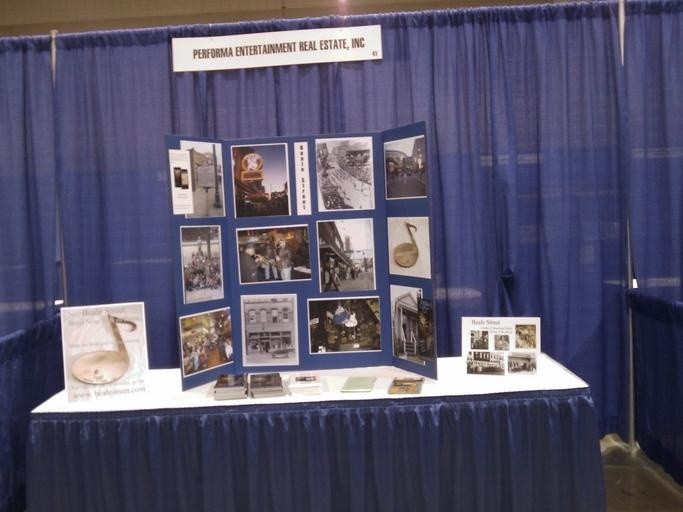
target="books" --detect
[387,377,424,395]
[288,373,320,396]
[341,375,376,392]
[249,371,285,398]
[213,374,248,400]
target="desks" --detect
[30,352,592,512]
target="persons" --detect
[239,244,263,282]
[224,338,233,360]
[277,240,292,280]
[189,344,202,372]
[257,239,278,281]
